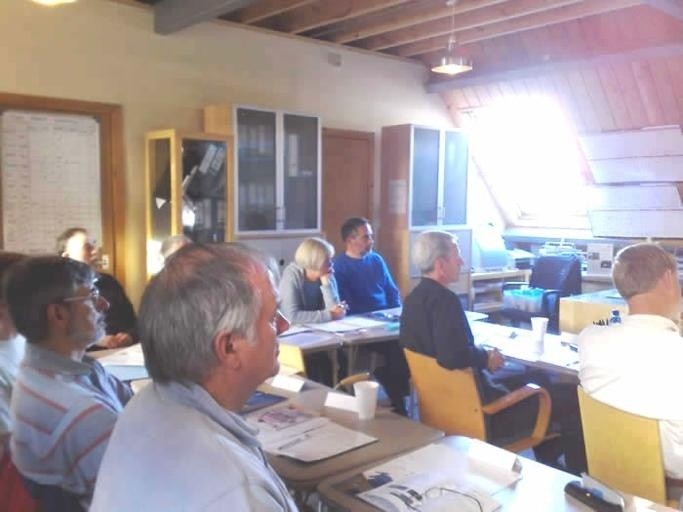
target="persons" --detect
[159,233,193,260]
[278,236,350,395]
[5,254,134,512]
[577,242,682,504]
[56,227,139,351]
[334,216,409,419]
[399,230,585,476]
[0,249,27,461]
[83,243,299,512]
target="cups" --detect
[530,317,550,342]
[354,382,380,421]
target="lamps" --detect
[428,2,474,75]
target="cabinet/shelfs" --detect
[377,123,472,319]
[143,101,323,293]
[471,268,530,329]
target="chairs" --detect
[497,253,580,334]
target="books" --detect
[360,443,523,497]
[302,315,385,332]
[238,121,300,214]
[155,143,226,244]
[357,470,503,512]
[245,400,379,463]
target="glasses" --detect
[61,286,99,304]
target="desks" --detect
[557,270,628,334]
